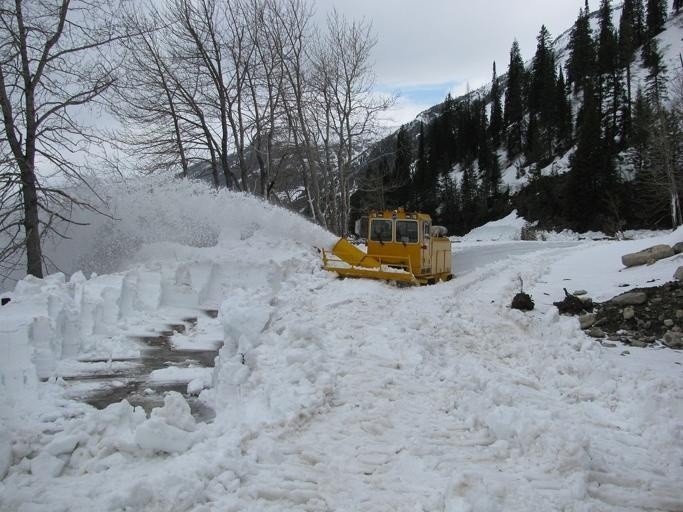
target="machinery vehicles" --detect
[320,205,453,288]
